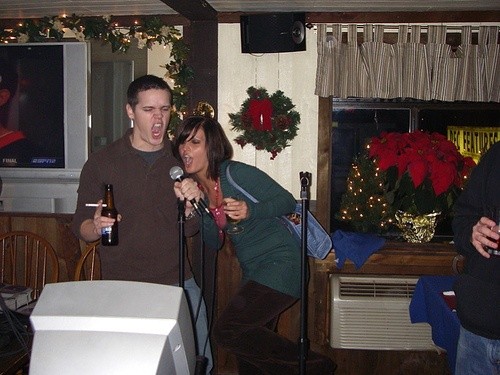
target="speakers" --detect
[240,11,306,54]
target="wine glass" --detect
[222,195,245,235]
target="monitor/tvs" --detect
[29,280,197,375]
[0,42,95,217]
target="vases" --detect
[394,210,441,244]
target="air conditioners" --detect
[327,273,445,352]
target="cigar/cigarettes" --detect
[85,203,107,207]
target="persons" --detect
[70,75,213,375]
[171,115,337,375]
[450,140,500,375]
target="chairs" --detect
[0,230,60,298]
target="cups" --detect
[484,206,500,256]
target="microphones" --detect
[170,166,204,216]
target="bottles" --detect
[101,183,120,246]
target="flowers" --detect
[0,13,196,137]
[368,131,477,217]
